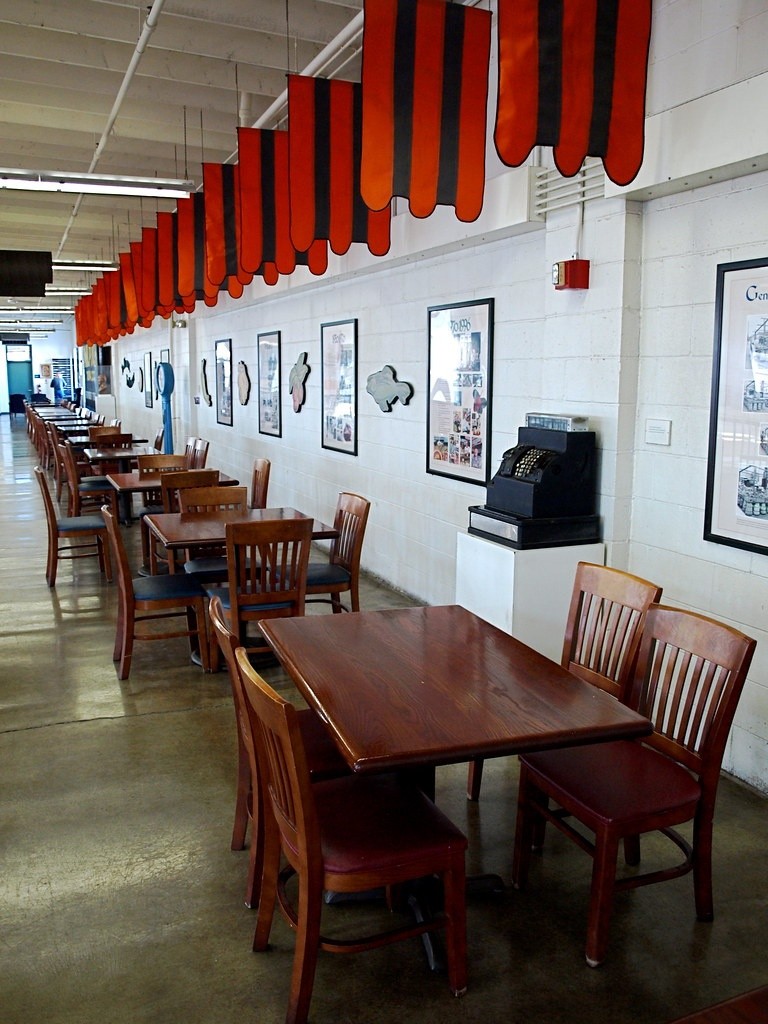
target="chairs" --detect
[205,594,384,910]
[558,562,664,695]
[22,396,370,681]
[524,608,757,967]
[234,646,469,1024]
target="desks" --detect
[41,416,84,421]
[84,448,164,479]
[105,473,239,528]
[45,420,96,427]
[58,426,102,433]
[66,435,149,449]
[257,603,654,931]
[140,508,340,602]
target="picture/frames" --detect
[214,339,234,426]
[160,349,170,365]
[426,298,495,487]
[703,256,768,556]
[144,352,153,408]
[257,331,282,438]
[322,318,358,456]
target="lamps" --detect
[0,168,196,197]
[0,258,121,332]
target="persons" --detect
[760,431,765,448]
[433,331,486,469]
[49,371,68,404]
[760,380,764,392]
[762,466,768,488]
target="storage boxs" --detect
[85,365,111,395]
